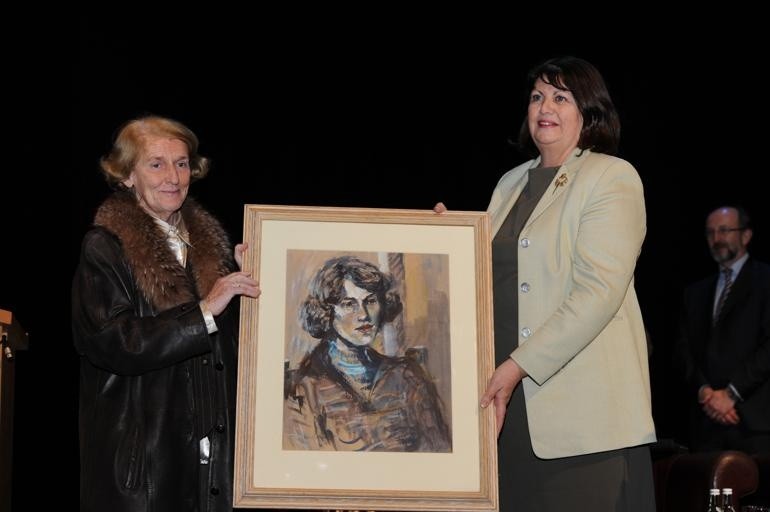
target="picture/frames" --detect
[232,204,501,507]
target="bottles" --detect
[1,334,13,359]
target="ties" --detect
[713,269,733,325]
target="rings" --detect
[236,282,241,288]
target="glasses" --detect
[707,228,742,235]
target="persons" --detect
[70,115,261,512]
[295,259,453,451]
[435,54,660,512]
[688,206,770,451]
